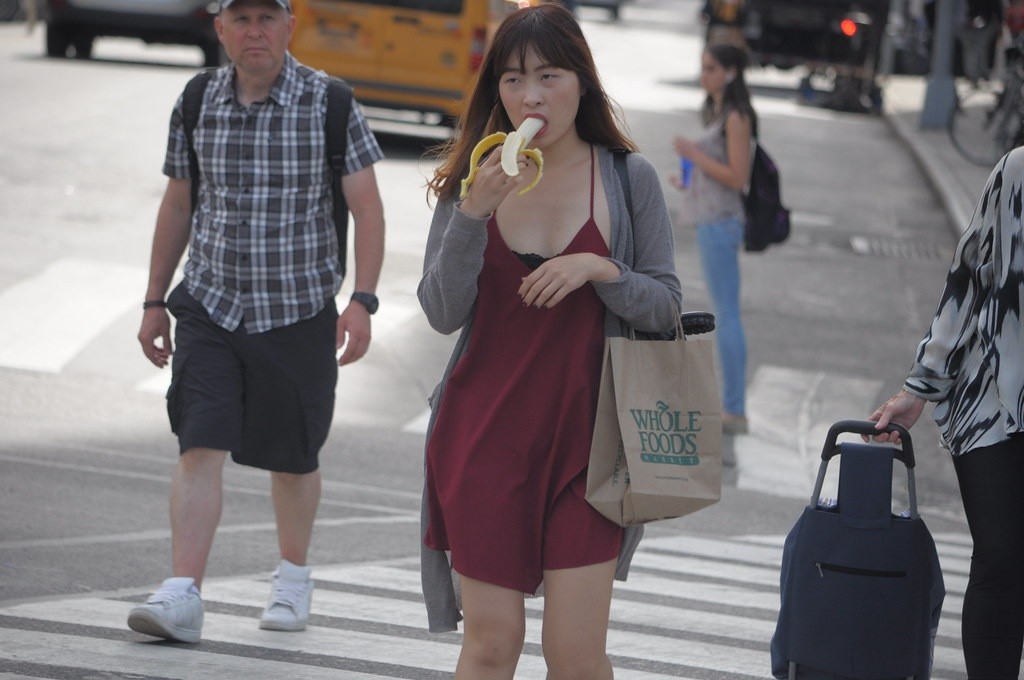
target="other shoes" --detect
[713,404,747,437]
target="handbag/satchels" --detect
[584,286,720,527]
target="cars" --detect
[47,0,227,67]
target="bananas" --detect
[458,117,546,199]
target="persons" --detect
[416,4,682,680]
[673,42,759,435]
[128,0,385,645]
[864,147,1024,680]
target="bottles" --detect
[679,154,694,188]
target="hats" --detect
[215,0,290,16]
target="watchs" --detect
[352,292,379,314]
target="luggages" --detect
[771,419,945,680]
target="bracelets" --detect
[143,301,168,309]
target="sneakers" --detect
[127,577,203,643]
[260,563,312,632]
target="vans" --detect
[287,0,520,116]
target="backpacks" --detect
[728,110,790,250]
